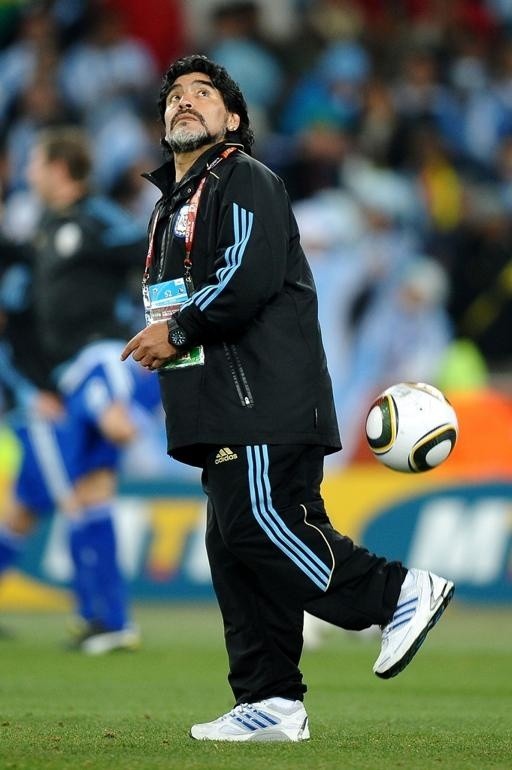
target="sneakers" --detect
[371,567,456,680]
[186,695,312,743]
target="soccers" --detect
[365,382,459,473]
